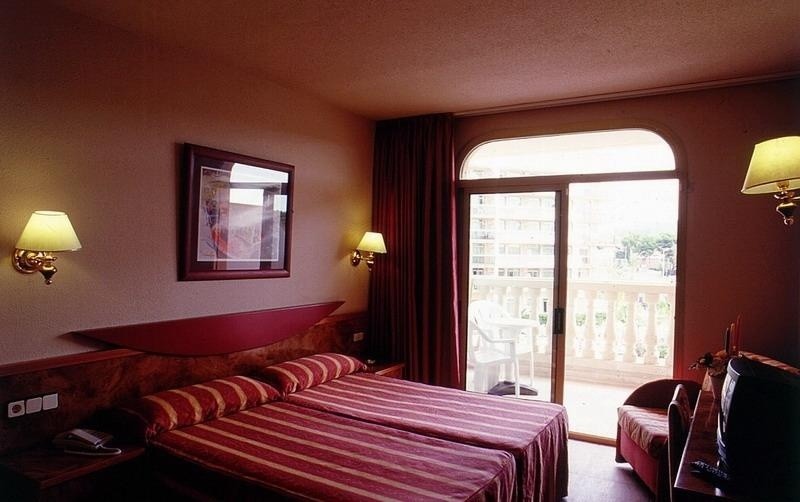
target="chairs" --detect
[668,384,692,502]
[467,299,538,396]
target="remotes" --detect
[689,460,731,489]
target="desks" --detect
[674,372,737,502]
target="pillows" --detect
[258,352,367,393]
[138,375,280,433]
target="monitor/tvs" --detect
[716,357,794,494]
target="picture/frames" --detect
[177,142,297,281]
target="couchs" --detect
[615,347,797,501]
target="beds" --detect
[130,366,569,502]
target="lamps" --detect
[740,134,800,228]
[349,230,388,271]
[12,209,83,286]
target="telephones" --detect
[55,426,122,458]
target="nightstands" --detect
[366,361,409,381]
[18,432,145,502]
